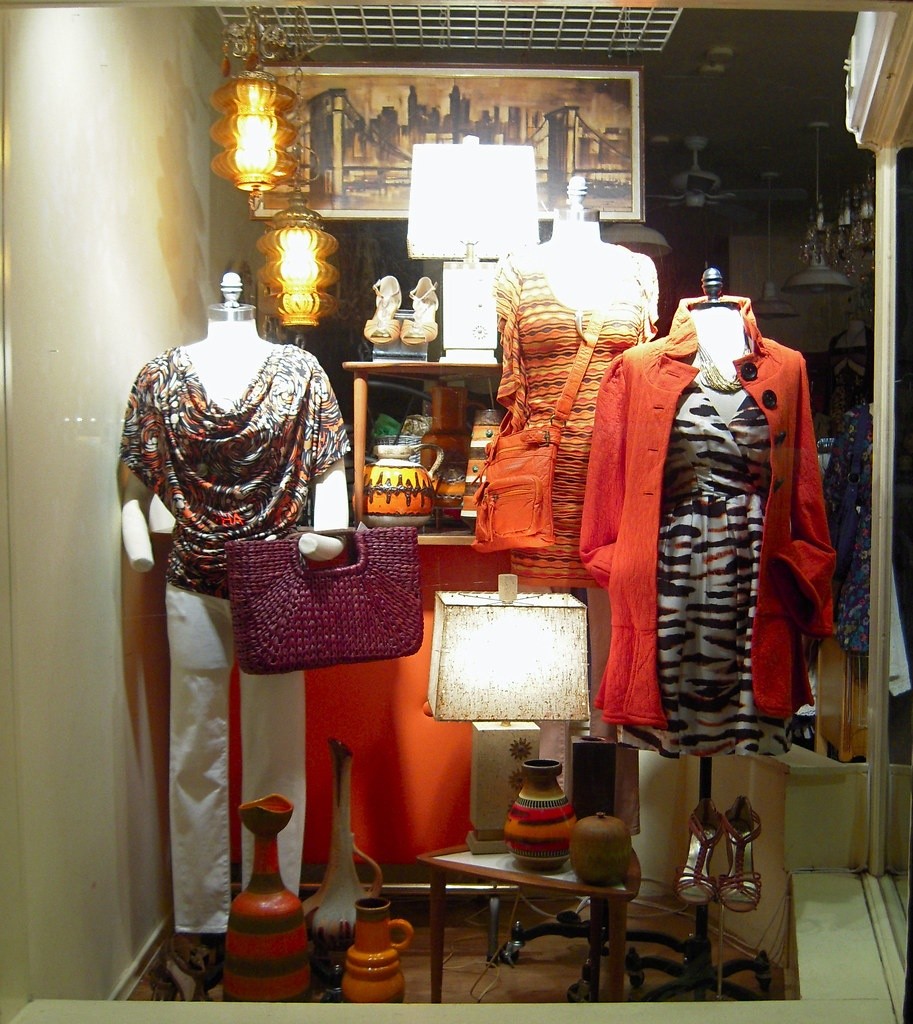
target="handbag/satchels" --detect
[225,529,425,677]
[471,425,562,552]
[826,405,869,582]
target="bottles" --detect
[505,760,577,871]
[342,897,411,1002]
[223,794,312,1003]
[298,737,383,998]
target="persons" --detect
[823,403,873,655]
[491,212,662,798]
[114,305,352,936]
[822,322,874,431]
[578,296,837,758]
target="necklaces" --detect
[696,343,750,394]
[845,328,865,347]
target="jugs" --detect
[353,445,444,529]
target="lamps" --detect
[428,573,589,854]
[798,165,875,296]
[600,222,673,258]
[212,5,306,212]
[780,120,856,295]
[256,136,340,328]
[751,169,799,320]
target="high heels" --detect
[363,275,401,345]
[718,795,764,913]
[400,276,442,347]
[675,799,723,906]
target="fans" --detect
[646,132,807,209]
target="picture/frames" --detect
[255,60,647,224]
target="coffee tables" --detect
[417,844,642,1001]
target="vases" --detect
[223,738,414,1004]
[503,760,576,867]
[350,385,505,532]
